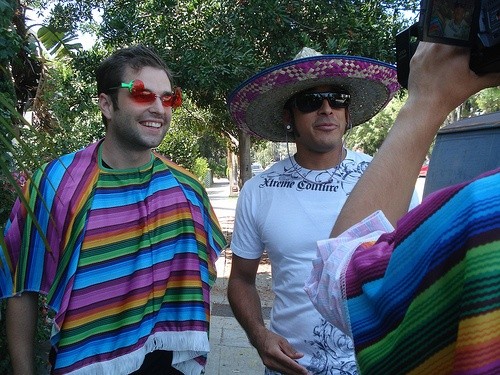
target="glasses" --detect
[296,90,351,114]
[103,80,183,108]
[455,4,464,8]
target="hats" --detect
[227,47,401,143]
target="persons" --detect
[428,0,447,38]
[354,145,364,152]
[304,40,500,375]
[0,44,229,375]
[227,47,420,375]
[443,1,471,39]
